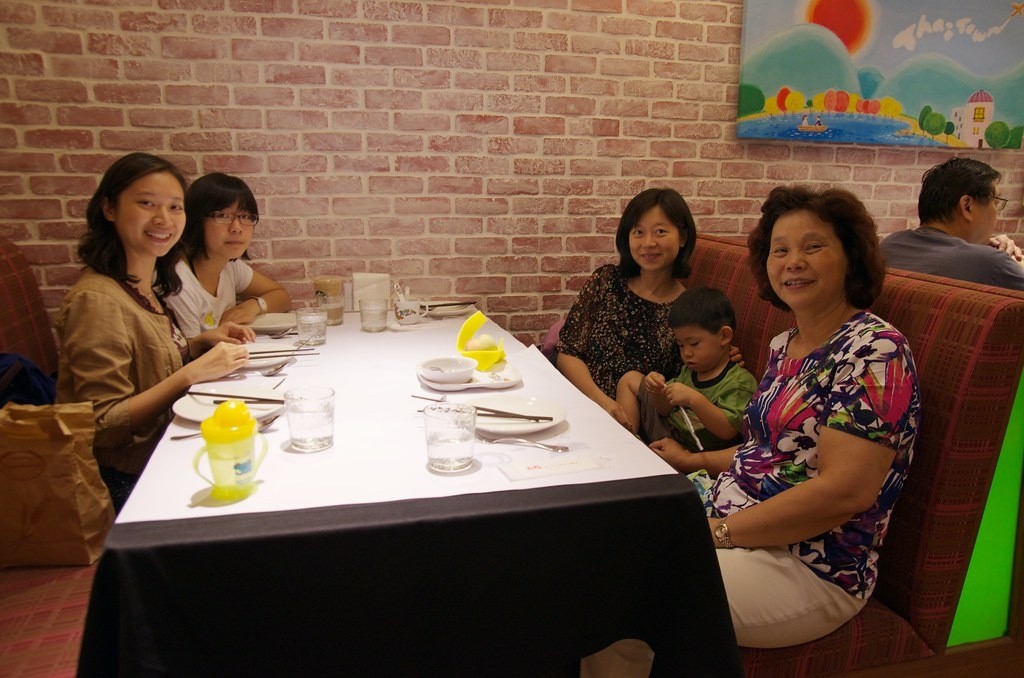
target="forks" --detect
[255,327,293,339]
[238,362,289,377]
[475,433,569,453]
[170,414,280,441]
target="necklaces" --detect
[136,285,160,306]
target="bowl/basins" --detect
[419,354,479,383]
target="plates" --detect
[442,395,566,440]
[415,360,523,391]
[246,343,297,370]
[172,384,288,423]
[249,312,297,334]
[420,300,476,317]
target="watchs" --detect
[248,297,267,314]
[715,517,735,548]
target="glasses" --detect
[203,209,259,226]
[990,191,1010,216]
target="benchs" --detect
[679,233,1024,678]
[0,237,101,678]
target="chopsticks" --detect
[249,348,321,361]
[411,395,553,422]
[184,390,285,404]
[421,301,478,309]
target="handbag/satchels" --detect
[0,401,113,565]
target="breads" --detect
[466,333,499,352]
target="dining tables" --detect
[69,291,739,678]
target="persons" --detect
[880,158,1024,291]
[153,172,292,344]
[581,183,921,678]
[556,187,745,432]
[616,287,757,480]
[54,152,256,497]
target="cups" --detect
[285,385,336,454]
[423,401,477,473]
[359,298,388,333]
[317,295,344,326]
[313,275,345,297]
[393,297,429,325]
[192,398,269,501]
[297,306,328,347]
